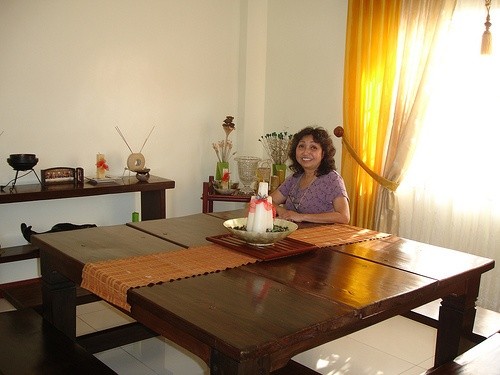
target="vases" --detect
[272,164,286,188]
[235,155,261,193]
[216,162,229,188]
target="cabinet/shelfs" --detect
[0,173,175,264]
[203,176,279,216]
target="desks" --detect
[32,209,495,375]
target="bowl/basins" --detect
[215,188,235,195]
[212,180,239,195]
[222,217,298,246]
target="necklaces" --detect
[290,171,318,210]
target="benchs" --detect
[400,296,500,340]
[0,277,104,310]
[0,310,118,375]
[417,333,500,375]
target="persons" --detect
[247,126,350,224]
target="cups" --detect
[271,176,279,192]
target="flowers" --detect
[213,116,237,162]
[259,131,293,165]
